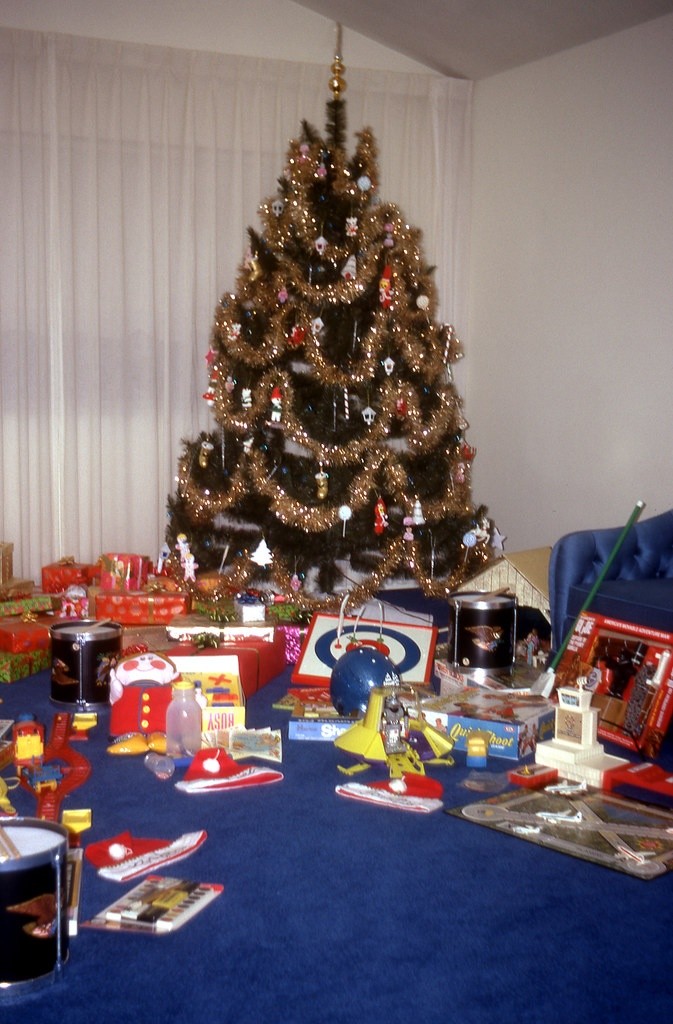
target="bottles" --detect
[166,682,202,757]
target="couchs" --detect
[549,510,673,671]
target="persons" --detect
[181,553,199,583]
[174,535,191,563]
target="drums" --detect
[50,618,124,710]
[1,815,70,992]
[445,588,518,683]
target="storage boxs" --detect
[0,535,673,763]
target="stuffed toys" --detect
[108,650,208,754]
